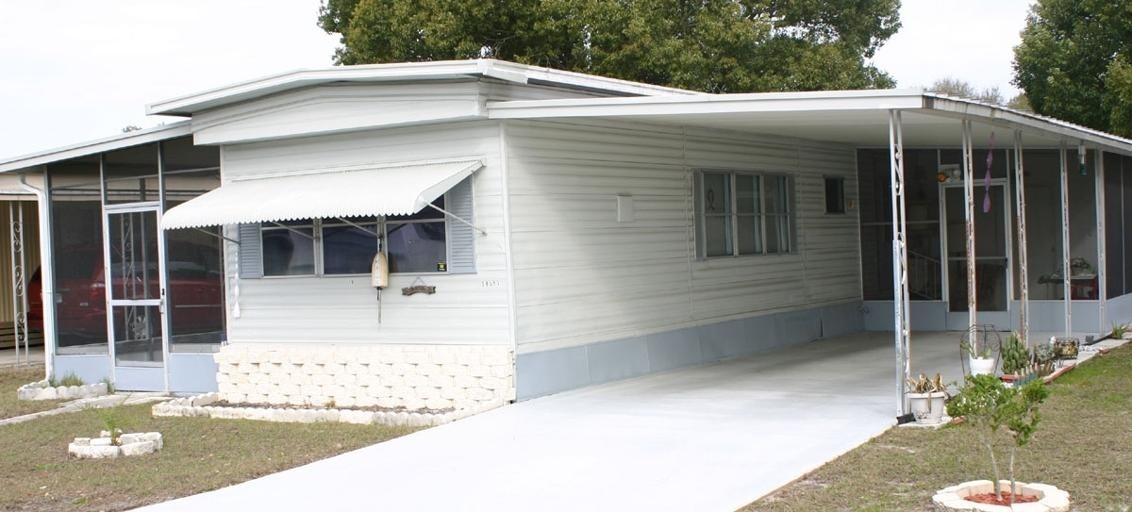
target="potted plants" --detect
[931,373,1071,512]
[1000,330,1031,383]
[959,324,1002,388]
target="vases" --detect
[903,391,946,424]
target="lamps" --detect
[1078,145,1087,175]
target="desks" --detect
[1038,271,1096,299]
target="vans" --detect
[27,236,225,353]
